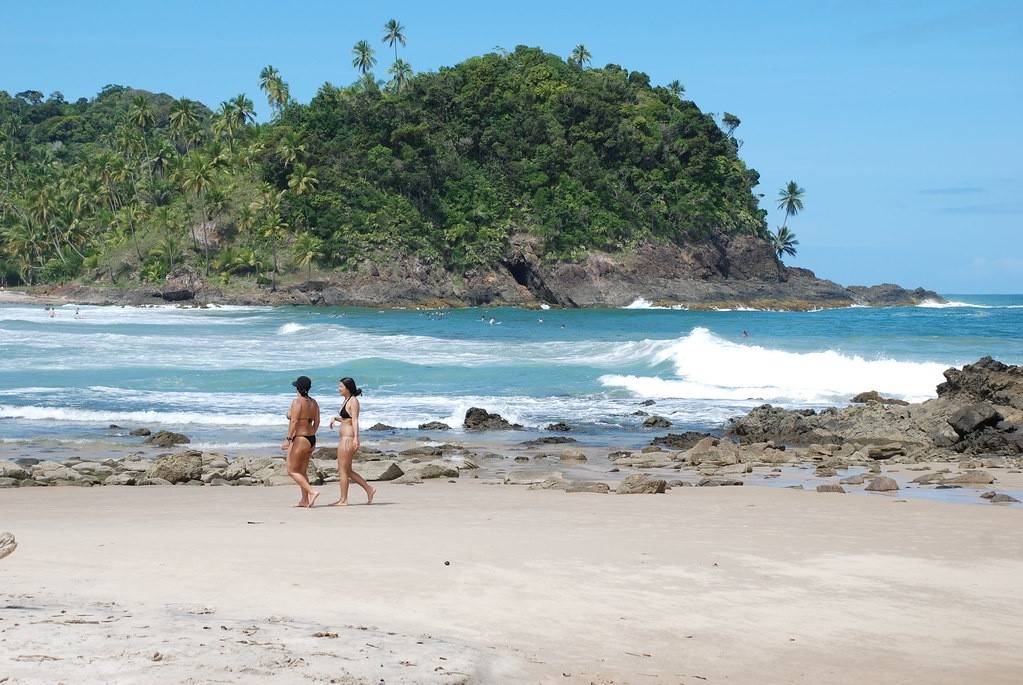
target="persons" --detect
[490,317,495,323]
[281,375,320,508]
[743,331,748,336]
[328,378,376,506]
[482,316,484,321]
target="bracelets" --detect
[286,436,292,440]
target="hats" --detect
[292,376,311,388]
